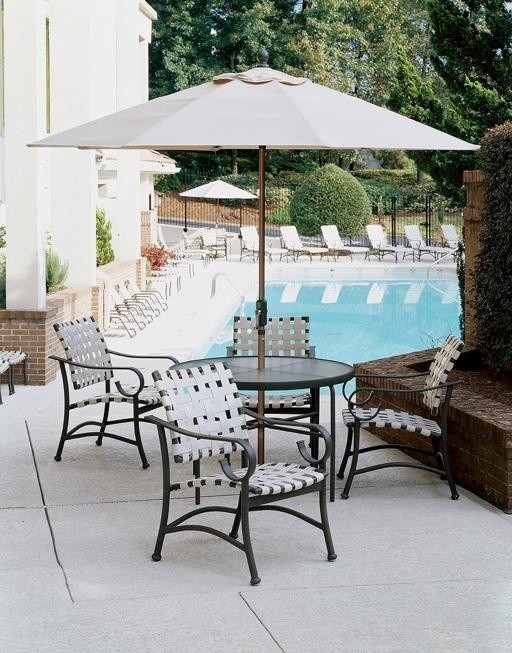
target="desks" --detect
[168,355,355,505]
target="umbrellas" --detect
[178,176,258,254]
[23,65,482,464]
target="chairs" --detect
[279,280,456,307]
[1,350,27,395]
[101,221,234,338]
[47,315,180,468]
[239,224,463,263]
[145,361,337,586]
[225,316,316,466]
[337,334,464,500]
